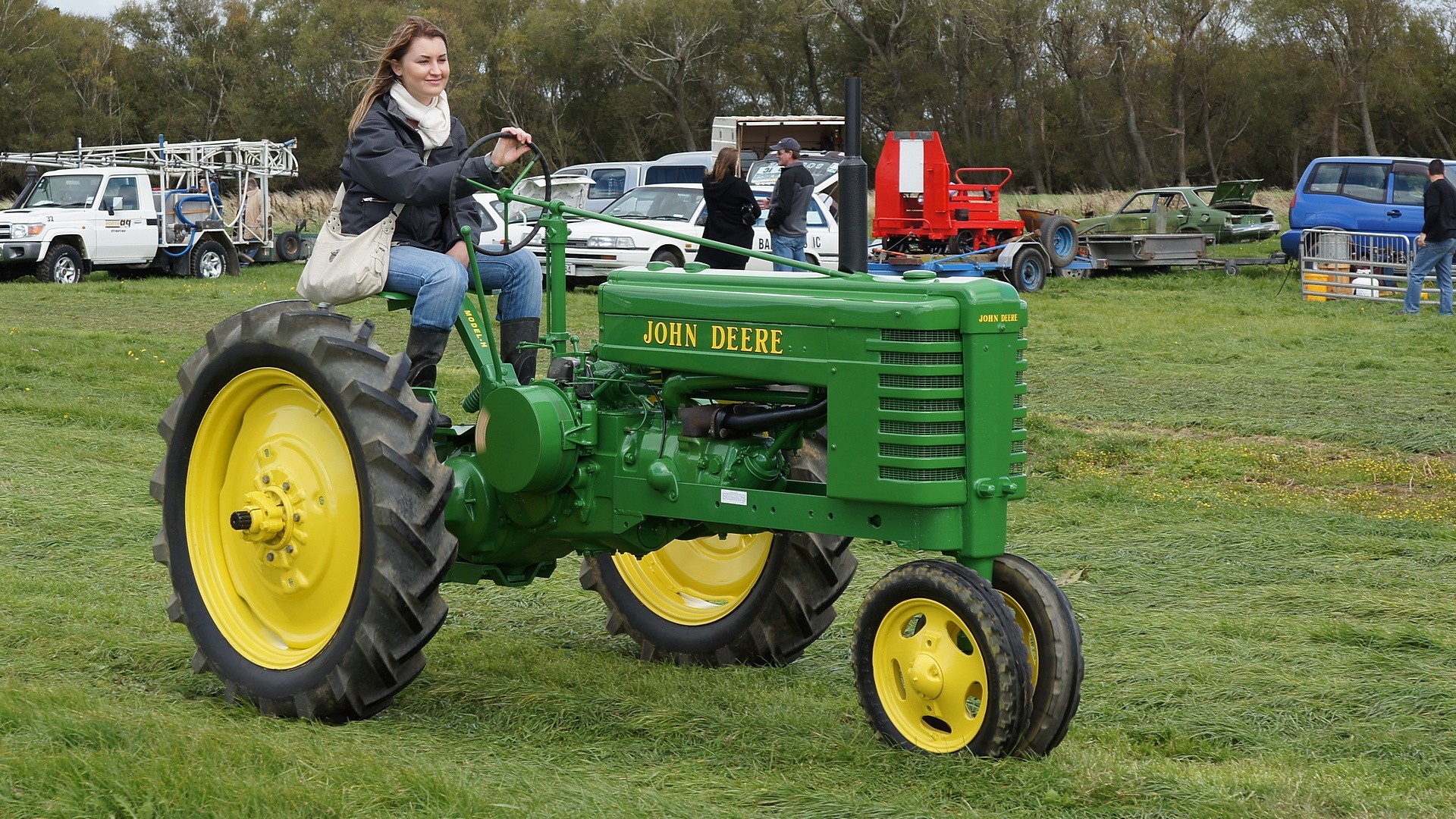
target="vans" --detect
[1279,156,1456,269]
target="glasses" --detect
[778,150,783,155]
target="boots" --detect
[500,317,540,385]
[406,325,452,428]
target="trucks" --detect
[0,166,242,284]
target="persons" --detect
[754,136,815,272]
[325,13,543,402]
[1397,159,1456,318]
[693,147,761,270]
[200,178,220,205]
[232,177,270,267]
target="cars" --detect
[468,174,596,250]
[524,147,846,300]
[1071,178,1282,246]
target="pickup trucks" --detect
[549,152,711,218]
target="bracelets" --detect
[484,151,504,173]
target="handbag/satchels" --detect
[741,203,755,226]
[296,216,395,305]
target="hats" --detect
[769,138,800,152]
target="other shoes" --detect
[1397,309,1418,317]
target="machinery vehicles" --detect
[867,127,1079,294]
[146,128,1085,762]
